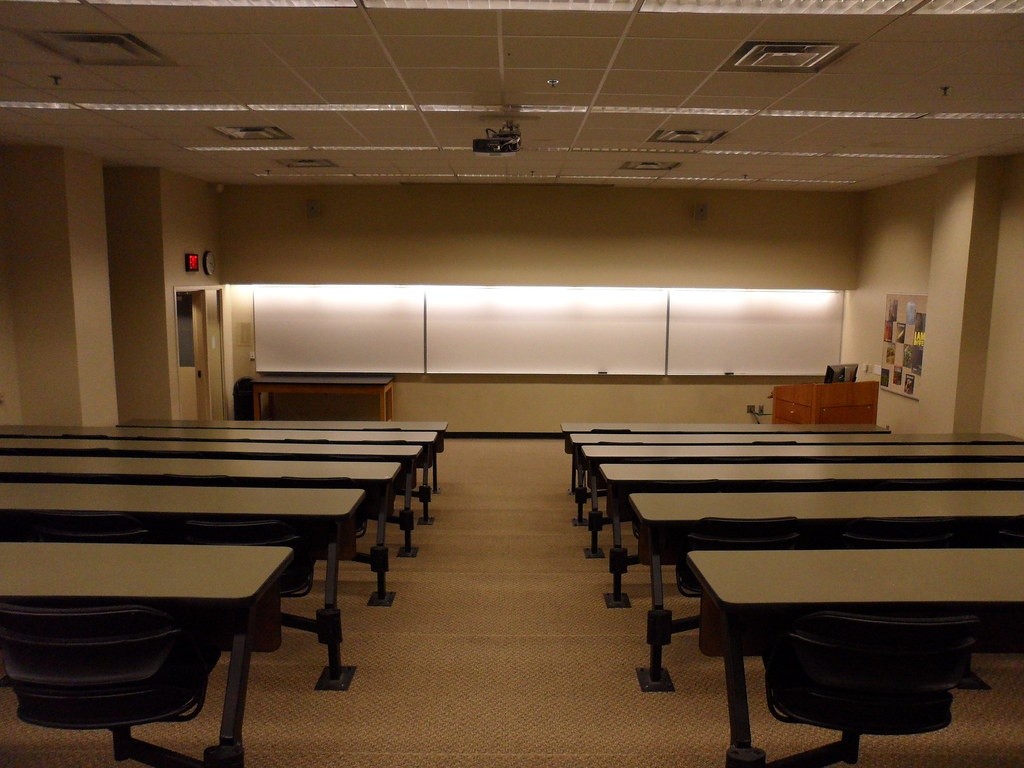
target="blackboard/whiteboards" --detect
[253,284,844,376]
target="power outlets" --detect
[747,405,755,413]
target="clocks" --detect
[202,251,215,276]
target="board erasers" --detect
[598,372,608,375]
[725,373,734,375]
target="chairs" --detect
[983,478,1024,491]
[34,510,150,542]
[757,610,981,768]
[0,603,220,768]
[676,513,803,598]
[994,513,1024,549]
[630,477,720,539]
[838,516,962,550]
[762,477,837,492]
[882,478,951,492]
[186,519,315,599]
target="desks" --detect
[560,419,1024,768]
[249,376,394,422]
[0,422,449,768]
